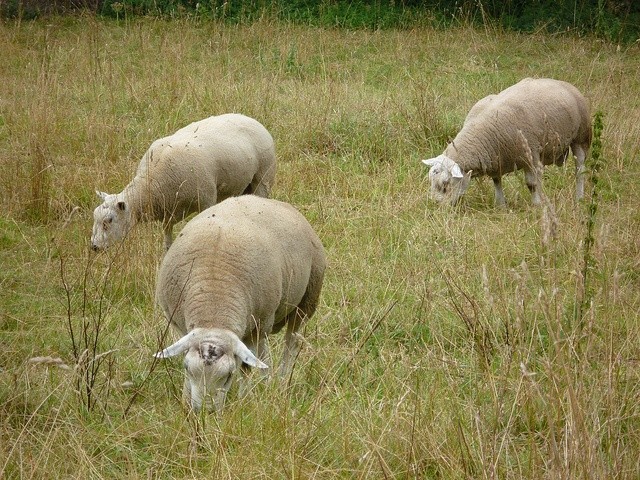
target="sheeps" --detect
[90,114,277,252]
[422,76,592,210]
[152,195,327,417]
